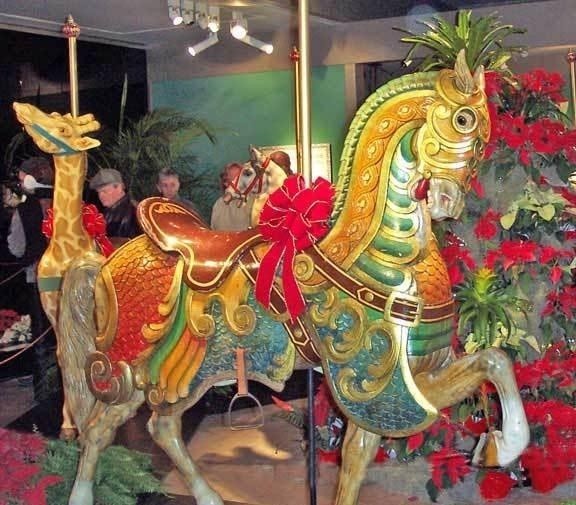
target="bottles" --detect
[17,157,50,175]
[88,167,122,188]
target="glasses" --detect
[170,3,246,58]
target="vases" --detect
[309,66,573,502]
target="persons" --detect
[270,150,294,178]
[210,159,255,232]
[157,164,198,213]
[90,166,143,239]
[0,151,63,432]
